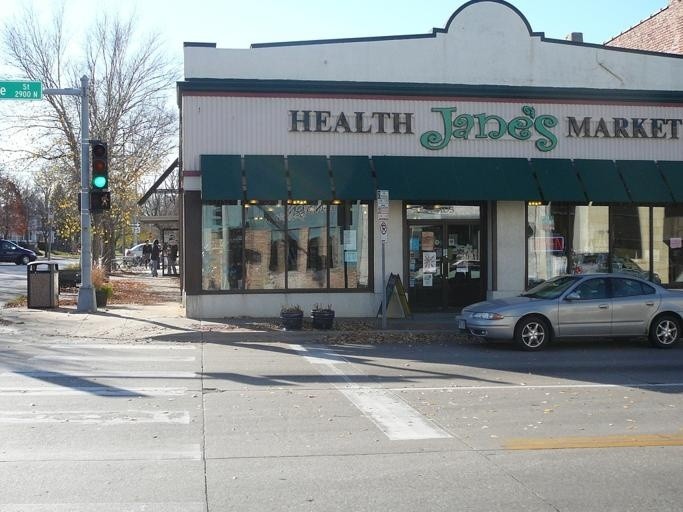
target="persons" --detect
[142,239,152,269]
[165,237,178,278]
[150,240,162,277]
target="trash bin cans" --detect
[27,261,59,308]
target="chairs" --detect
[579,283,605,299]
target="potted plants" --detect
[95,284,113,308]
[279,301,334,331]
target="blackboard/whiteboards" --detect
[378,272,398,315]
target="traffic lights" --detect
[91,139,113,214]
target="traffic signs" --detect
[1,81,40,99]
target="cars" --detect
[455,273,683,352]
[0,239,36,265]
[125,242,173,262]
